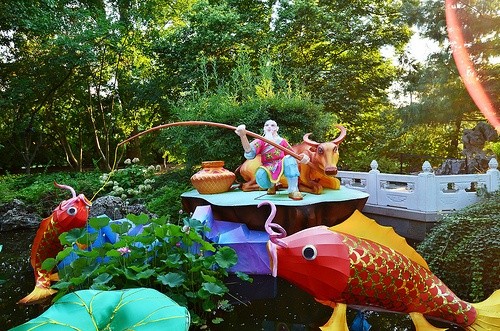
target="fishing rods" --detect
[118,121,320,172]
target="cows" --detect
[229,125,347,195]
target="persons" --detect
[234,119,311,200]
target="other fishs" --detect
[256,201,500,331]
[15,181,93,305]
